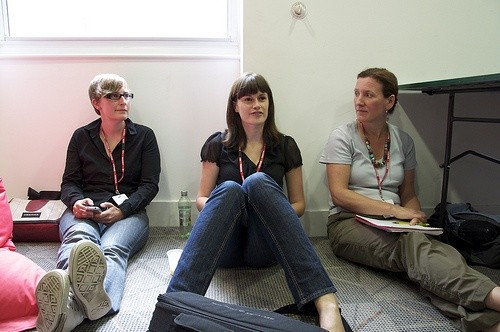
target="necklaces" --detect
[360,123,389,166]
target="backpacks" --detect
[427,202,500,268]
[146,292,354,332]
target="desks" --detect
[398,72,500,201]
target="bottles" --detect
[178,191,192,239]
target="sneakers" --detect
[36,239,112,332]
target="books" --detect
[355,214,443,236]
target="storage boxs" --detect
[8,197,67,242]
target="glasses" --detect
[104,92,133,101]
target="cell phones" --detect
[86,206,101,213]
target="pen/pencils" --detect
[392,220,430,226]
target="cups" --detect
[166,249,183,275]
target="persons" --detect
[319,67,500,332]
[34,74,161,332]
[165,73,345,332]
